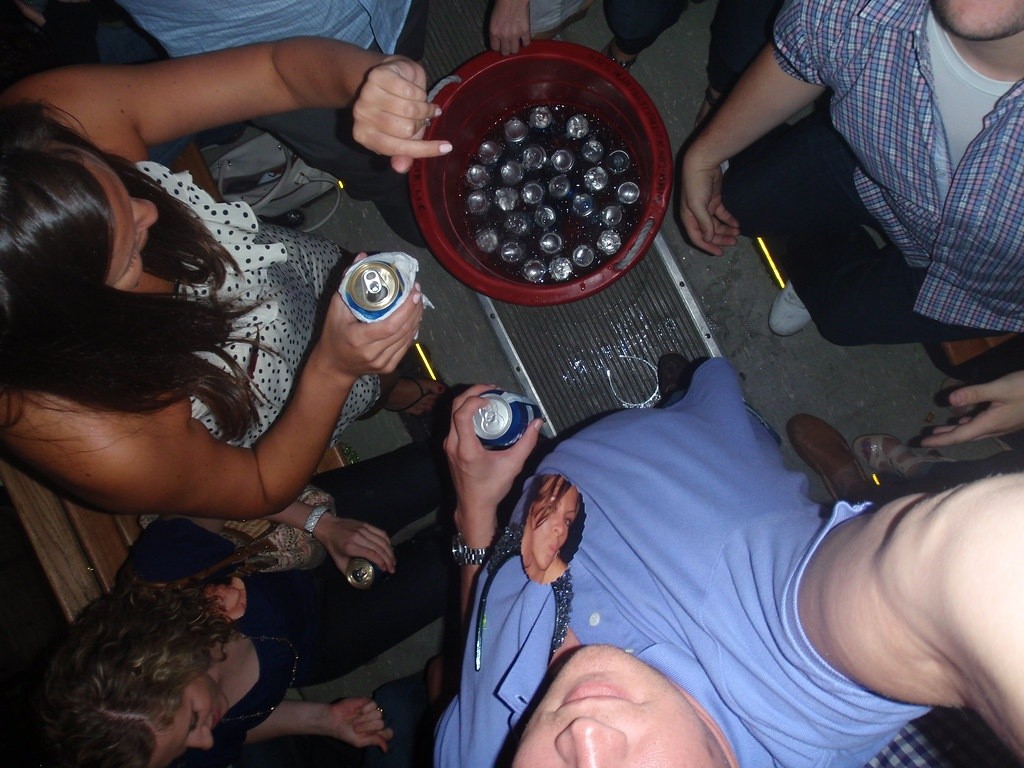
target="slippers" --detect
[383,376,447,418]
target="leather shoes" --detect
[788,414,875,504]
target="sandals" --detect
[600,36,637,73]
[853,434,957,484]
[693,85,726,131]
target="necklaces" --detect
[218,631,300,722]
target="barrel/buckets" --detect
[408,38,675,308]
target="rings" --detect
[375,707,385,713]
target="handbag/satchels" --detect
[208,132,340,232]
[130,484,337,598]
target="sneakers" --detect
[768,280,814,336]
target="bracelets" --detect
[303,504,337,536]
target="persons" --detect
[2,1,1023,768]
[519,473,586,583]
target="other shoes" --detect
[196,125,246,151]
[258,208,304,228]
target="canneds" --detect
[463,107,640,282]
[346,262,404,319]
[346,557,380,589]
[472,390,542,448]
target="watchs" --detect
[451,533,490,567]
